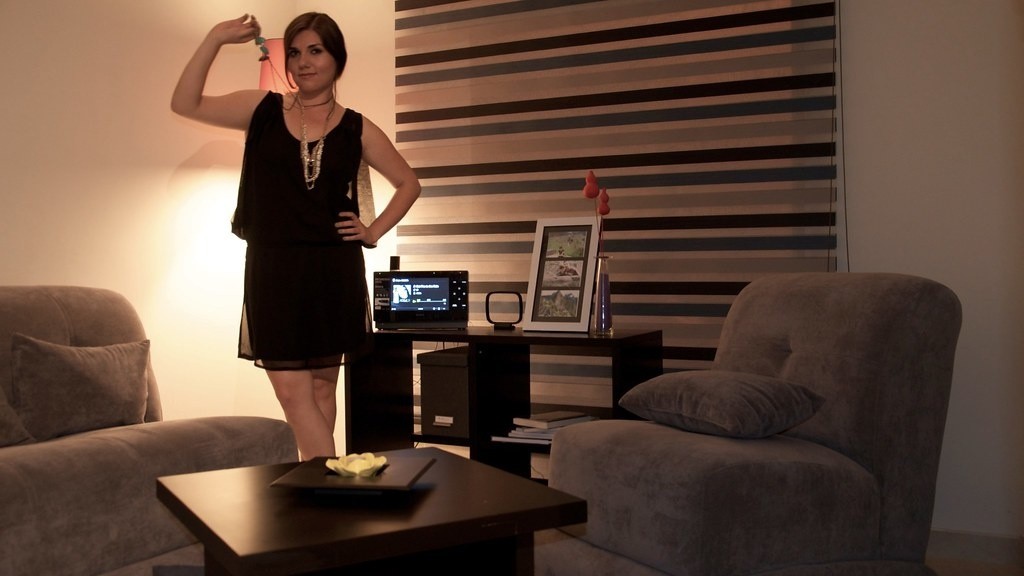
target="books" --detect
[492,411,600,444]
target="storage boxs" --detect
[417,346,474,438]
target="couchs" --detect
[0,283,299,576]
[533,272,963,576]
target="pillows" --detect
[10,332,150,442]
[619,369,827,440]
[0,384,36,448]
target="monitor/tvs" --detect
[373,271,469,323]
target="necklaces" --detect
[300,106,335,189]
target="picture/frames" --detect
[522,215,602,333]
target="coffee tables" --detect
[154,447,590,576]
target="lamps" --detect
[258,38,299,93]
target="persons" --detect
[172,13,421,462]
[556,264,578,276]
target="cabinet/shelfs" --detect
[344,326,662,487]
